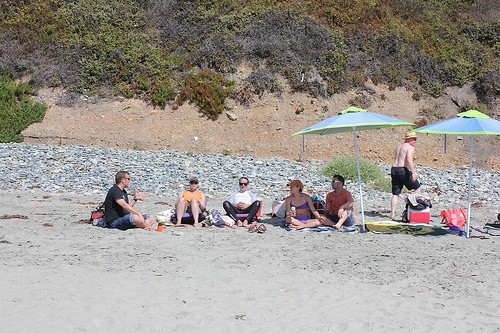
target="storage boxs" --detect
[407,205,431,224]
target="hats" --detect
[189,177,198,183]
[287,180,303,187]
[404,132,417,139]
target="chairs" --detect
[172,197,207,223]
[236,199,262,220]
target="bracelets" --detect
[133,198,137,201]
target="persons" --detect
[174,176,206,228]
[283,180,326,230]
[104,171,157,230]
[223,177,262,227]
[391,132,422,221]
[320,174,355,230]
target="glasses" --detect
[190,181,198,185]
[239,182,248,187]
[125,177,131,181]
[332,180,340,183]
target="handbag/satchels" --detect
[440,208,467,229]
[89,209,108,229]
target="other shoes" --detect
[248,224,258,233]
[258,224,266,233]
[406,194,424,211]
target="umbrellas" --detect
[290,105,415,232]
[410,108,500,238]
